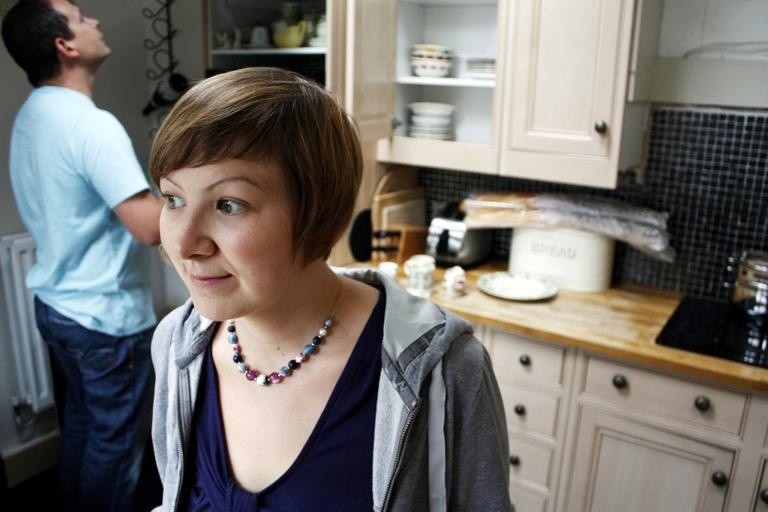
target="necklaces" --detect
[226,279,345,387]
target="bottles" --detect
[141,73,190,116]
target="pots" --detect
[724,251,768,326]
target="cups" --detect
[441,266,466,299]
[250,26,269,45]
[379,261,399,279]
[403,254,436,289]
[274,21,307,48]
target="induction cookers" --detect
[654,290,766,371]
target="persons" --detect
[1,0,161,512]
[148,67,516,512]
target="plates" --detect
[308,37,327,46]
[476,271,560,301]
[408,125,455,141]
[466,57,496,79]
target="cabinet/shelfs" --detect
[202,0,343,113]
[344,1,651,191]
[472,323,768,512]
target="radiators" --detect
[0,232,55,438]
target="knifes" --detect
[369,229,400,252]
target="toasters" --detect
[424,200,494,271]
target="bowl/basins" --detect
[407,102,456,128]
[410,42,455,77]
[316,23,327,37]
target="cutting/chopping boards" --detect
[370,170,427,267]
